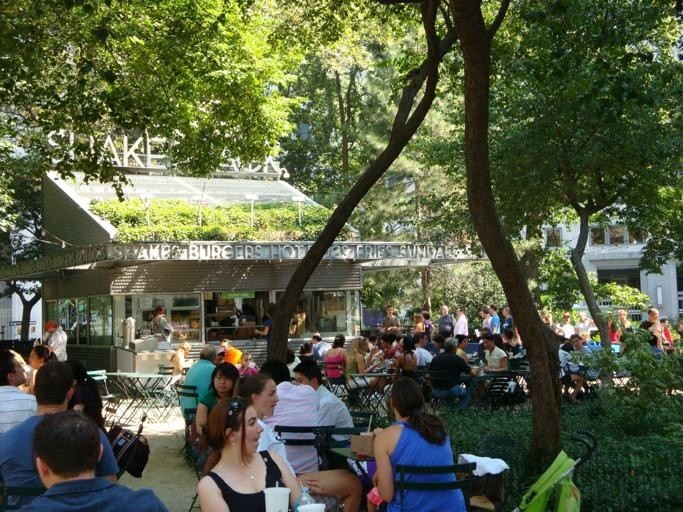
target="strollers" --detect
[510,428,599,510]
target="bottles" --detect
[294,486,314,512]
[248,363,255,368]
[479,359,488,376]
[350,432,374,459]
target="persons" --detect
[0,410,169,512]
[64,358,104,428]
[605,309,634,343]
[414,305,468,335]
[195,362,363,511]
[640,308,682,353]
[381,306,400,326]
[298,326,523,411]
[44,320,68,362]
[0,350,37,433]
[373,378,467,511]
[0,361,120,508]
[254,306,274,338]
[289,308,306,335]
[18,346,58,395]
[178,337,261,426]
[150,307,173,341]
[169,342,192,375]
[540,309,594,400]
[479,306,514,334]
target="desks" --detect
[461,343,483,354]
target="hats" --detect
[562,313,571,317]
[311,331,321,341]
[42,320,57,328]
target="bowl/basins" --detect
[209,322,222,327]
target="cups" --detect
[263,488,291,512]
[295,504,325,512]
[173,319,190,329]
[506,352,512,360]
[372,355,397,369]
[42,331,50,341]
[466,346,482,369]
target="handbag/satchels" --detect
[125,430,149,479]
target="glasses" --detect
[222,400,245,427]
